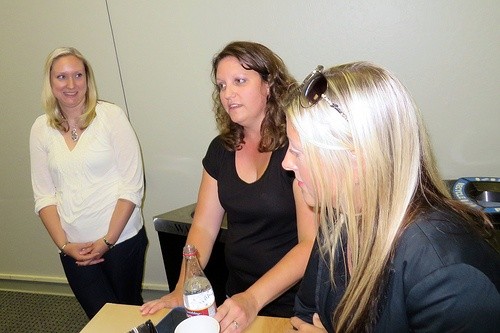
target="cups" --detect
[174,316,220,333]
[126,319,158,333]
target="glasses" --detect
[299,65,348,123]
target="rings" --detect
[233,321,239,329]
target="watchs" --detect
[58,242,72,256]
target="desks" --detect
[79,302,294,333]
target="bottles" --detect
[182,244,217,318]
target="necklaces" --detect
[59,112,82,141]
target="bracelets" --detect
[103,236,116,248]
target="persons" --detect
[139,42,320,333]
[30,47,148,320]
[282,61,500,333]
